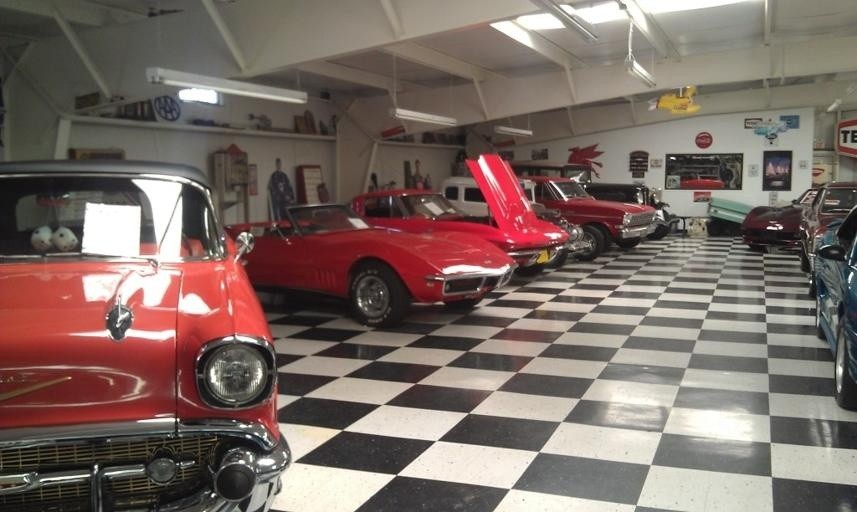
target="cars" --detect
[2,155,288,512]
[736,171,857,410]
[676,171,727,187]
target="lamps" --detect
[493,70,536,139]
[622,18,657,89]
[390,43,459,128]
[145,2,307,105]
